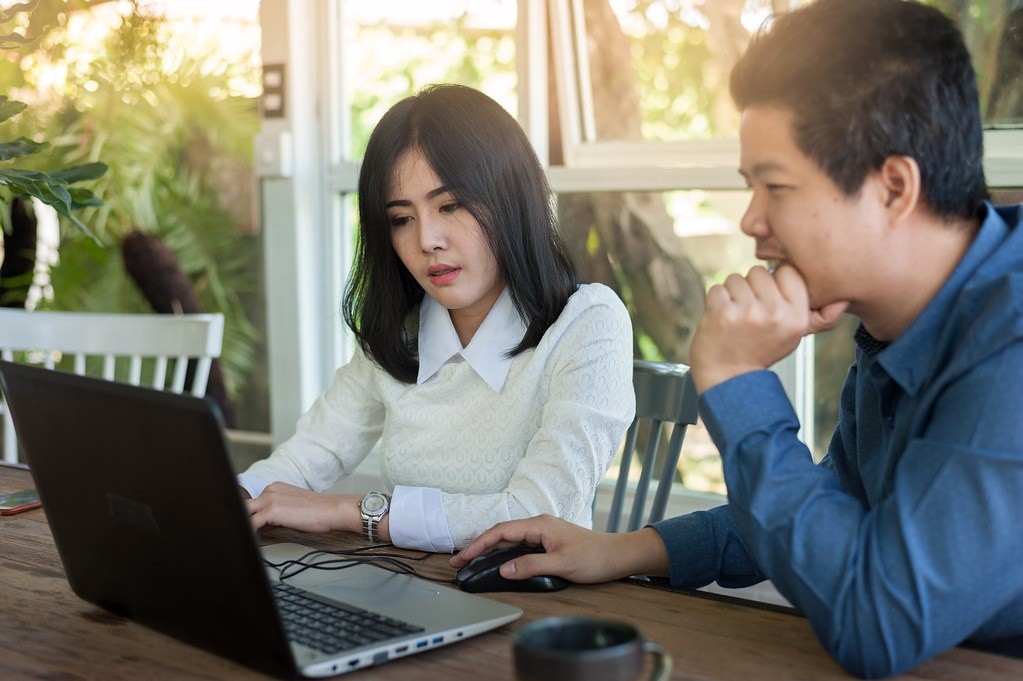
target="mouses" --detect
[456,544,568,594]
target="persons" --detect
[236,85,637,554]
[450,2,1021,681]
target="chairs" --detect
[0,302,225,476]
[591,354,699,582]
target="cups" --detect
[511,615,673,681]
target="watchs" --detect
[357,491,391,543]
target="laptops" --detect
[0,360,521,681]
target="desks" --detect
[0,459,1023,681]
